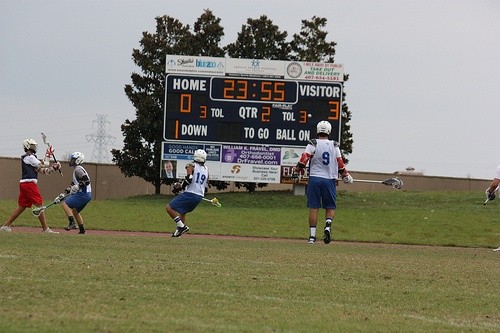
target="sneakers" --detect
[172,225,189,237]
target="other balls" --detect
[212,197,219,203]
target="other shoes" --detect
[323,227,331,244]
[64,222,76,231]
[42,227,59,233]
[2,225,11,230]
[308,236,316,243]
[78,232,85,234]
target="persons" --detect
[164,162,175,178]
[0,138,61,234]
[485,165,500,251]
[296,121,354,244]
[55,152,92,234]
[166,149,208,237]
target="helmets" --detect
[193,149,208,162]
[23,138,40,154]
[317,120,331,135]
[68,152,85,167]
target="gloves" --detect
[342,173,353,183]
[70,185,79,195]
[43,161,61,176]
[42,146,54,167]
[294,165,305,175]
[53,193,65,204]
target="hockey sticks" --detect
[483,186,500,205]
[301,170,404,190]
[42,132,63,177]
[177,188,223,207]
[32,193,70,217]
